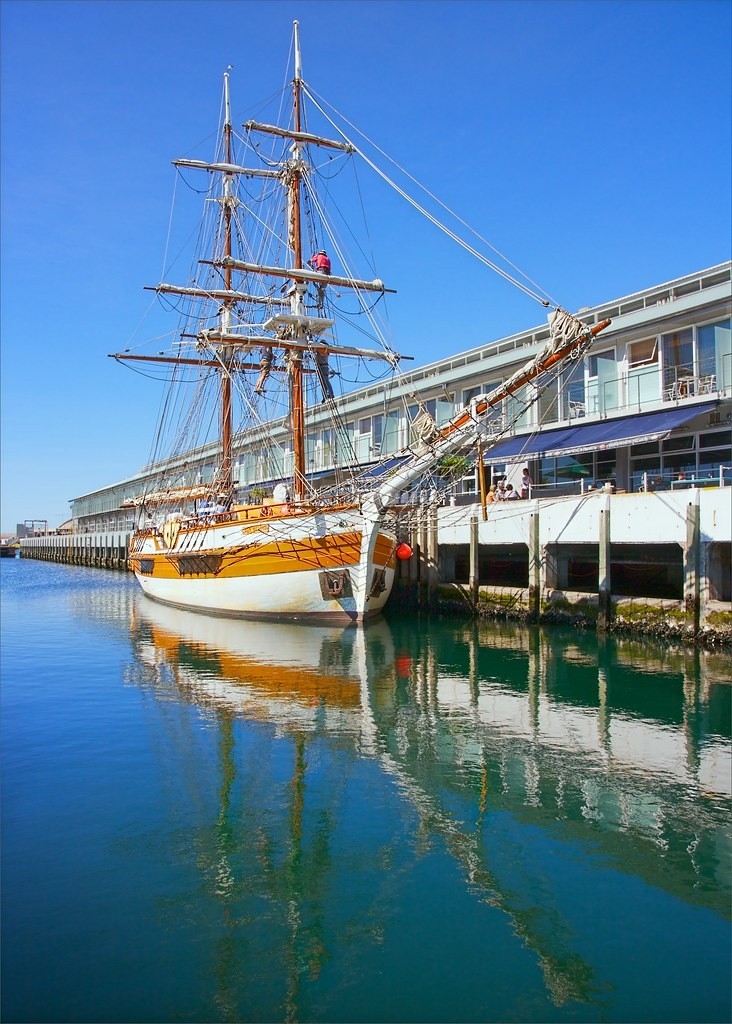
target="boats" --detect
[0,538,19,558]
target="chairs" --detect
[570,401,586,418]
[698,375,716,395]
[661,382,682,402]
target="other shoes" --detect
[253,388,267,393]
[321,395,334,404]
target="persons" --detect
[310,250,331,308]
[486,482,518,502]
[677,471,686,481]
[145,493,233,530]
[254,346,277,393]
[316,339,334,403]
[522,468,533,498]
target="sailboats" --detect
[127,592,399,918]
[99,19,611,633]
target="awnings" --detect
[354,404,720,481]
[238,471,334,491]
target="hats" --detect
[319,250,326,254]
